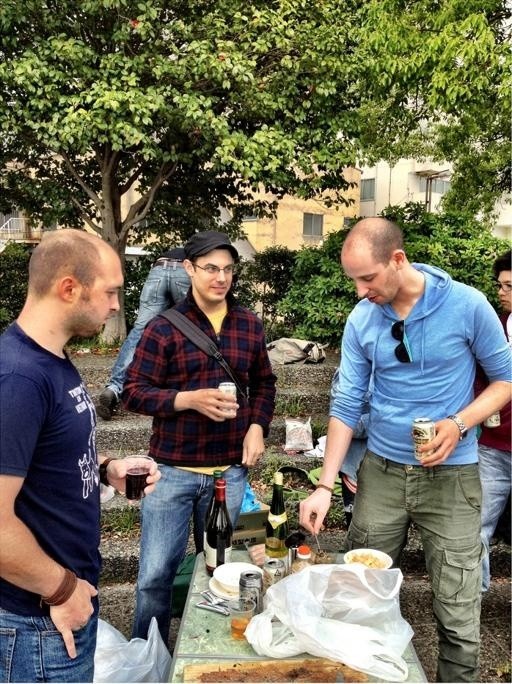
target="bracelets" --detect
[42,569,77,606]
[316,485,333,492]
[98,457,117,486]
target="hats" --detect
[183,230,239,262]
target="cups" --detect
[312,544,338,564]
[124,455,153,501]
[228,598,257,641]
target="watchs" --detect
[447,415,468,441]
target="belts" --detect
[152,258,182,270]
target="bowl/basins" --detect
[212,562,265,594]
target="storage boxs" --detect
[230,501,271,550]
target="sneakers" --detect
[95,387,118,420]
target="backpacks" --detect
[260,336,328,367]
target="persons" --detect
[0,228,162,683]
[299,218,512,683]
[96,248,191,421]
[473,250,512,597]
[121,230,278,650]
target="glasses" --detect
[186,263,238,276]
[390,319,412,363]
[493,284,512,293]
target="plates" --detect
[208,576,240,601]
[343,548,394,570]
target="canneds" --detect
[218,382,237,419]
[239,570,263,615]
[413,418,436,461]
[263,557,286,596]
[484,410,501,428]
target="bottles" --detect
[265,469,290,576]
[290,544,313,576]
[202,468,234,578]
